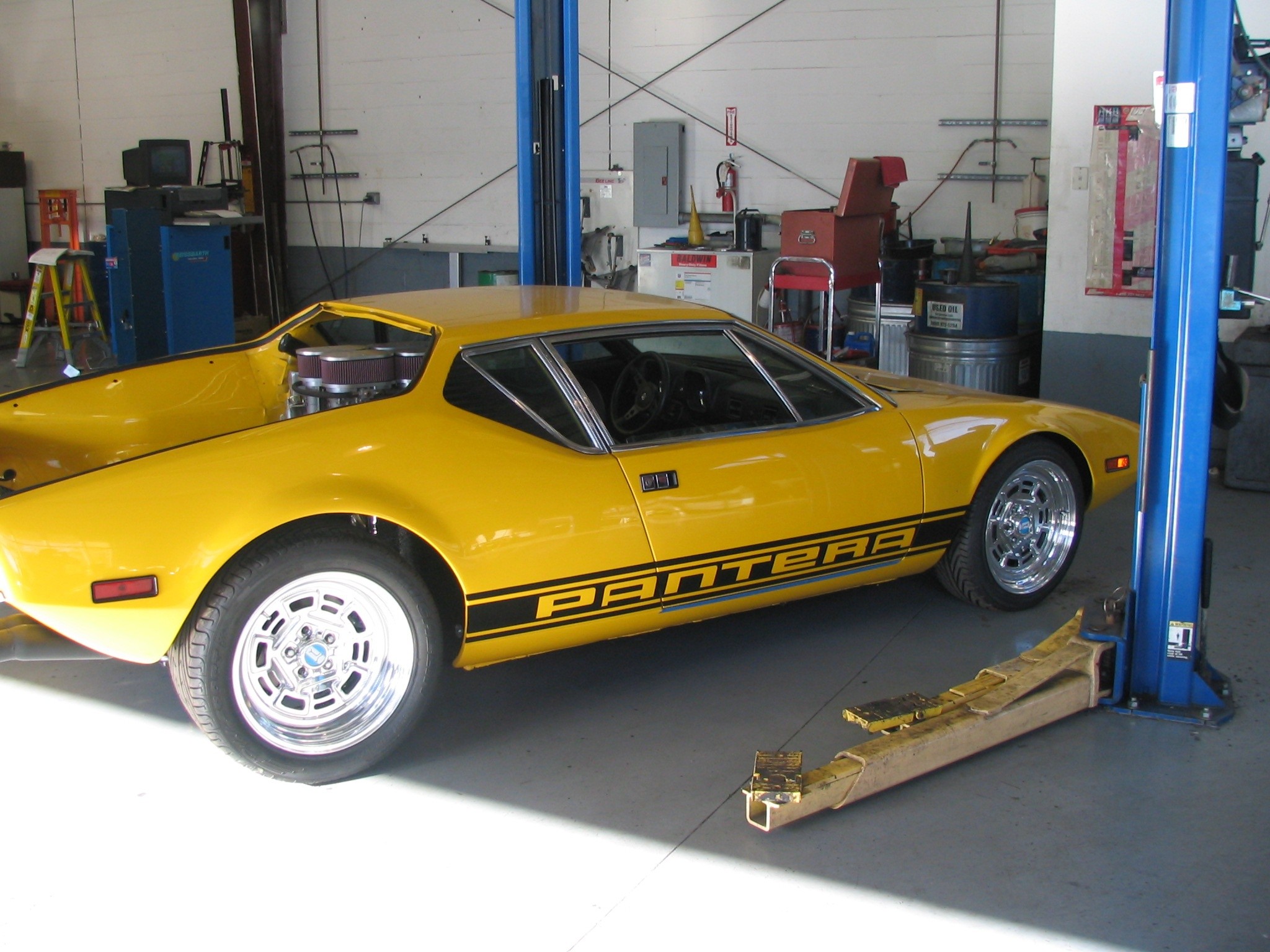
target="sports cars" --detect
[0,284,1139,785]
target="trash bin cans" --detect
[1224,323,1270,494]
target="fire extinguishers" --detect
[716,153,743,211]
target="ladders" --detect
[17,255,109,372]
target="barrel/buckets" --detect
[844,331,874,357]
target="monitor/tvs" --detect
[122,139,192,188]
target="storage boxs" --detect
[778,156,908,275]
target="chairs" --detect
[536,377,622,449]
